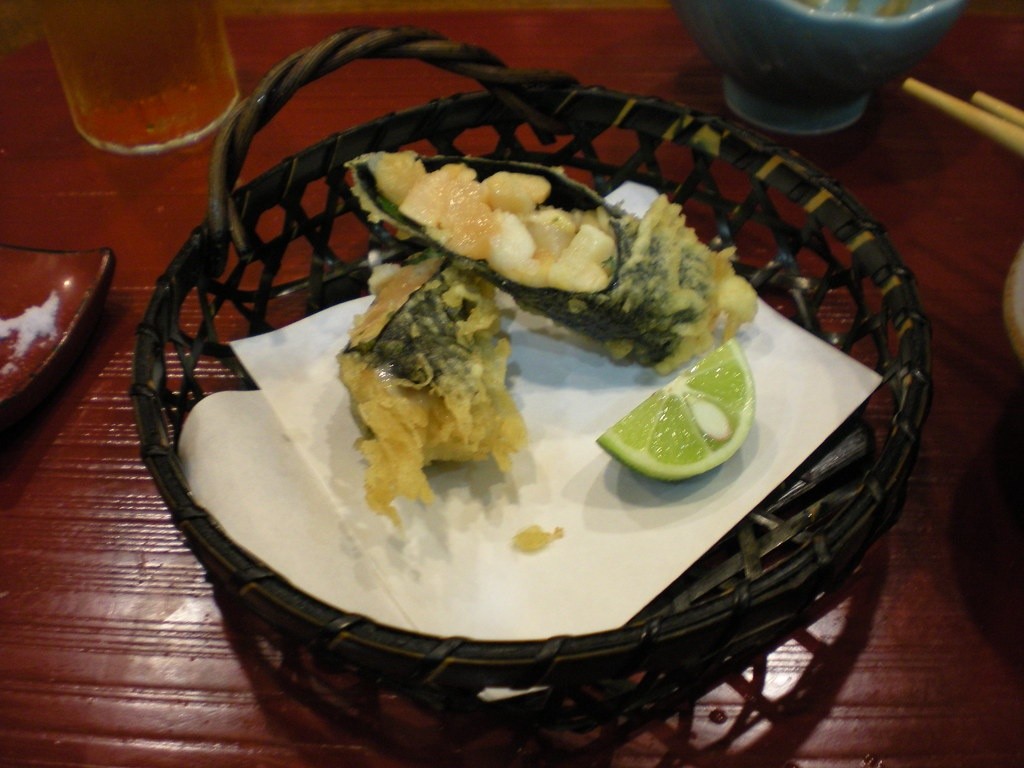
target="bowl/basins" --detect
[671,0,965,134]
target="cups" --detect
[37,0,238,153]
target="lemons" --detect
[597,338,755,481]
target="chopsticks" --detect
[902,78,1024,156]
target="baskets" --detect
[129,24,935,733]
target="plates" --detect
[0,243,115,422]
